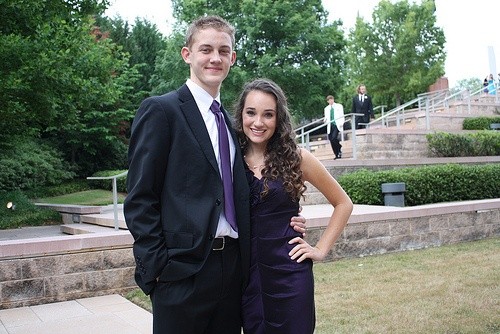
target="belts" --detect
[213,236,232,251]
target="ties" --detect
[210,100,239,233]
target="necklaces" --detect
[245,158,261,169]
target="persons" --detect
[232,79,353,334]
[352,84,375,130]
[323,95,344,160]
[124,15,308,334]
[483,73,500,95]
